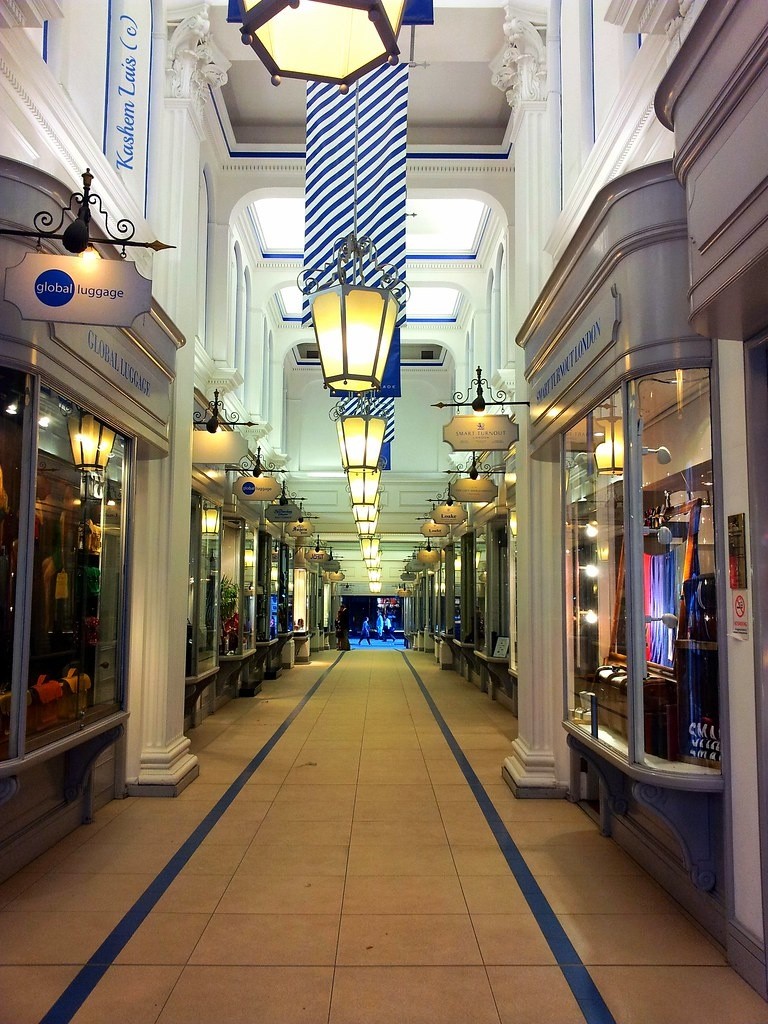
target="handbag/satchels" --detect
[390,626,395,632]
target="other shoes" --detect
[381,640,387,642]
[369,644,373,646]
[393,638,395,643]
[358,642,362,647]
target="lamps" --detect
[355,505,383,537]
[297,81,410,396]
[365,549,383,569]
[347,466,381,508]
[368,568,382,582]
[596,396,625,475]
[237,0,408,95]
[358,534,381,560]
[329,391,390,476]
[369,581,382,593]
[345,485,384,522]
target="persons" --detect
[294,619,304,630]
[335,612,343,651]
[339,604,350,651]
[382,614,396,643]
[358,617,372,647]
[376,612,383,640]
